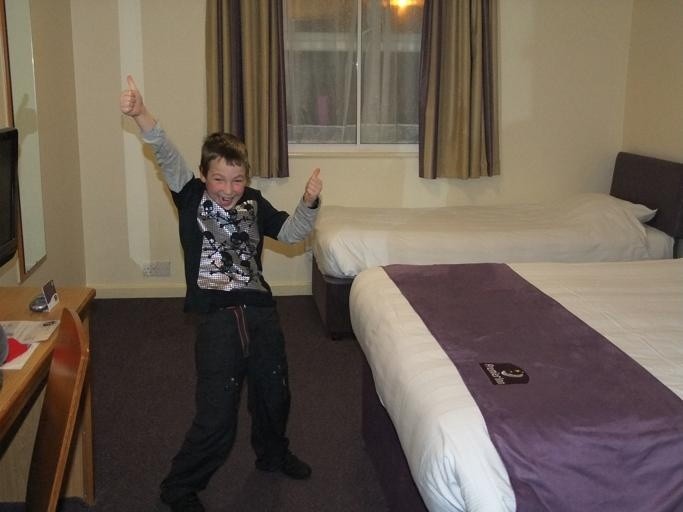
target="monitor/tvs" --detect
[0,127,18,267]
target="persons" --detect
[120,76,322,511]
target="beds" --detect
[348,257,683,512]
[313,150,683,339]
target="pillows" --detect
[608,194,657,225]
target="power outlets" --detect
[143,260,172,278]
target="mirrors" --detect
[0,0,45,284]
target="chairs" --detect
[26,305,90,512]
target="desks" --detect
[0,285,96,512]
